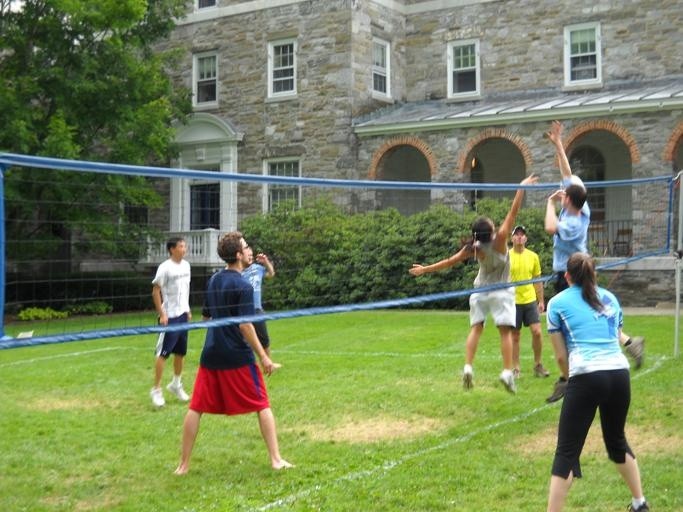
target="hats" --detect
[511,226,527,236]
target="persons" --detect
[172,232,292,474]
[508,225,550,381]
[150,238,189,407]
[241,247,283,369]
[545,251,650,511]
[409,171,540,395]
[545,120,644,404]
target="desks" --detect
[585,224,607,258]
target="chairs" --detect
[612,227,633,257]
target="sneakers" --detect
[627,501,648,512]
[166,382,189,402]
[150,386,165,406]
[462,364,474,391]
[534,364,550,378]
[500,374,517,396]
[625,337,645,371]
[514,367,520,379]
[545,379,568,403]
